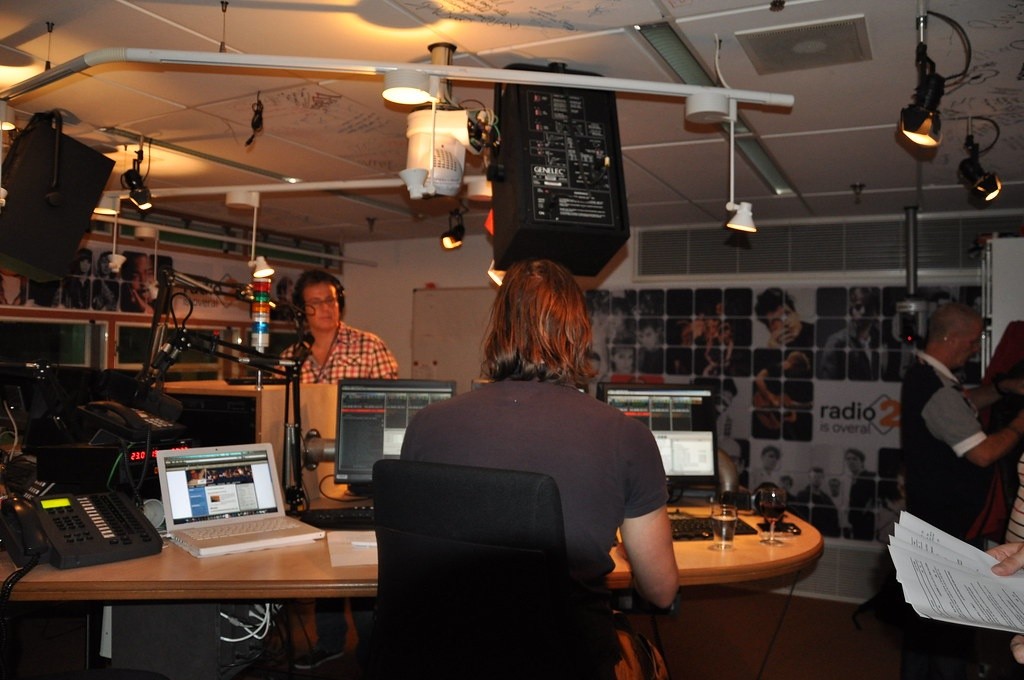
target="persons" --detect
[266,269,398,383]
[122,251,160,313]
[610,345,637,374]
[984,542,1024,663]
[899,301,1024,680]
[695,377,738,422]
[400,260,679,680]
[754,287,814,348]
[750,444,878,542]
[637,321,661,350]
[817,287,893,382]
[61,247,120,312]
[601,298,636,345]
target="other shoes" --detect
[293,650,344,668]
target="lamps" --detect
[120,136,152,210]
[441,200,469,249]
[226,190,275,277]
[685,94,757,233]
[957,116,1003,203]
[382,70,436,199]
[898,0,943,147]
[469,180,507,285]
[135,226,158,299]
[0,100,16,207]
[93,196,127,271]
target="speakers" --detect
[0,120,116,283]
[491,61,633,280]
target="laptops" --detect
[155,443,326,558]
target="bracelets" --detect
[995,382,1006,397]
[1008,426,1021,437]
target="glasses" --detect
[309,297,337,308]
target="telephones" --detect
[0,487,163,570]
[74,398,187,439]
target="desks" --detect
[0,445,826,602]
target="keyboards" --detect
[299,505,374,530]
[671,516,758,540]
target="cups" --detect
[710,504,738,550]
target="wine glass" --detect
[760,489,787,546]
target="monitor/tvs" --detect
[648,427,719,483]
[333,377,457,498]
